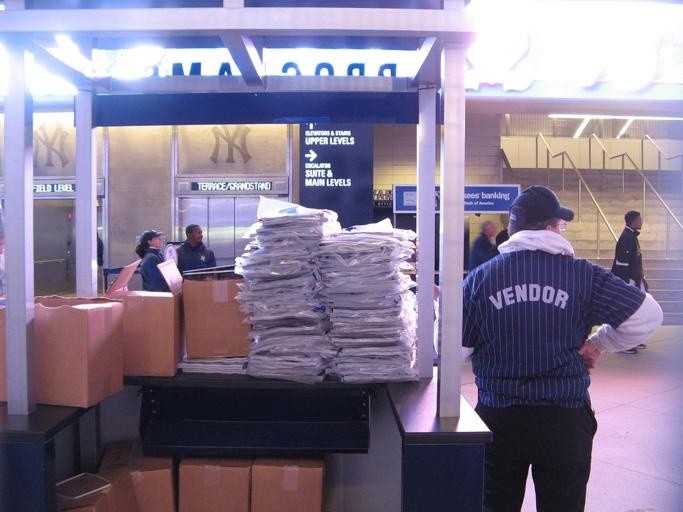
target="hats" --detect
[508,185,574,224]
[142,229,163,239]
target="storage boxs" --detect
[0,261,251,410]
[54,438,326,510]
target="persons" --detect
[462,185,663,512]
[610,211,647,354]
[135,229,168,292]
[468,221,509,272]
[175,224,216,281]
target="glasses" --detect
[551,221,566,231]
